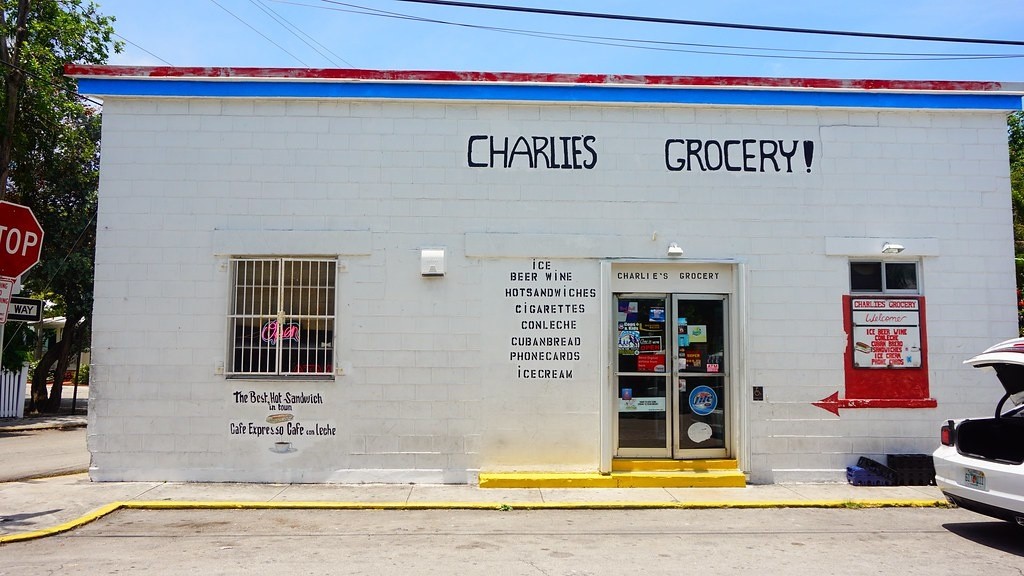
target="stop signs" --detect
[0,200,44,280]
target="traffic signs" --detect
[7,297,42,323]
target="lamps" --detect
[667,243,683,256]
[881,242,905,254]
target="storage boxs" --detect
[846,454,937,487]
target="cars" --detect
[932,337,1024,531]
